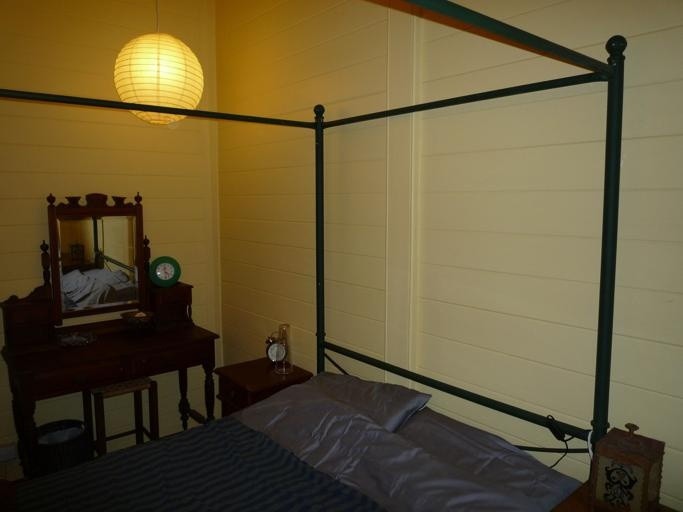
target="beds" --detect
[60,214,133,308]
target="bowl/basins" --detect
[120,310,154,326]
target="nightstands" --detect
[0,372,600,512]
[210,356,310,420]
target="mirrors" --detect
[34,187,151,321]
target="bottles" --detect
[276,323,291,374]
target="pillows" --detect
[314,366,577,508]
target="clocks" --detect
[149,255,181,286]
[264,329,289,366]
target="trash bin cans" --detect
[35,419,95,474]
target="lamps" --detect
[111,0,204,127]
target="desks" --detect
[0,283,223,479]
